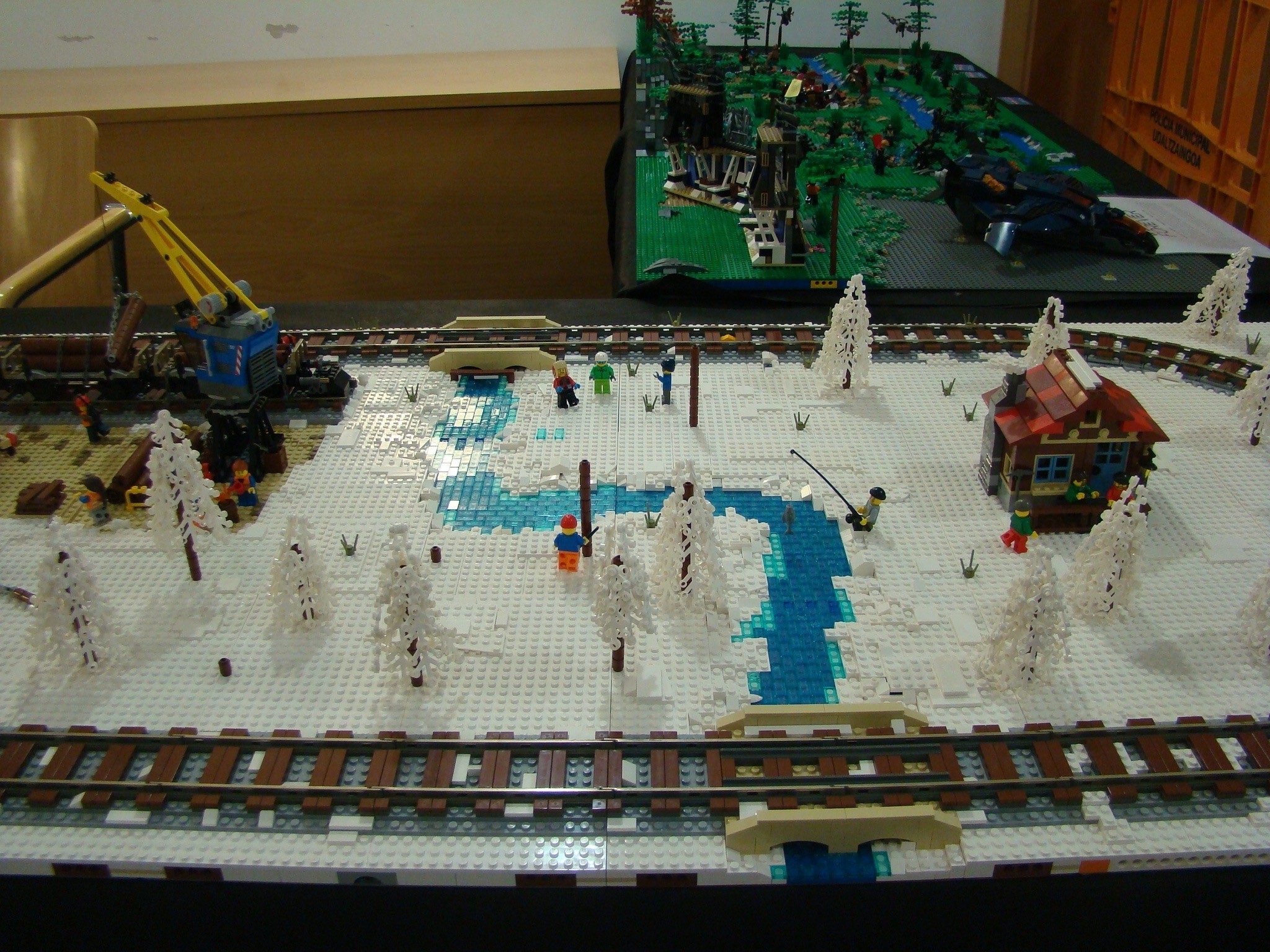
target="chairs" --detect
[0,113,100,309]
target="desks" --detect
[610,44,1270,301]
[0,44,623,309]
